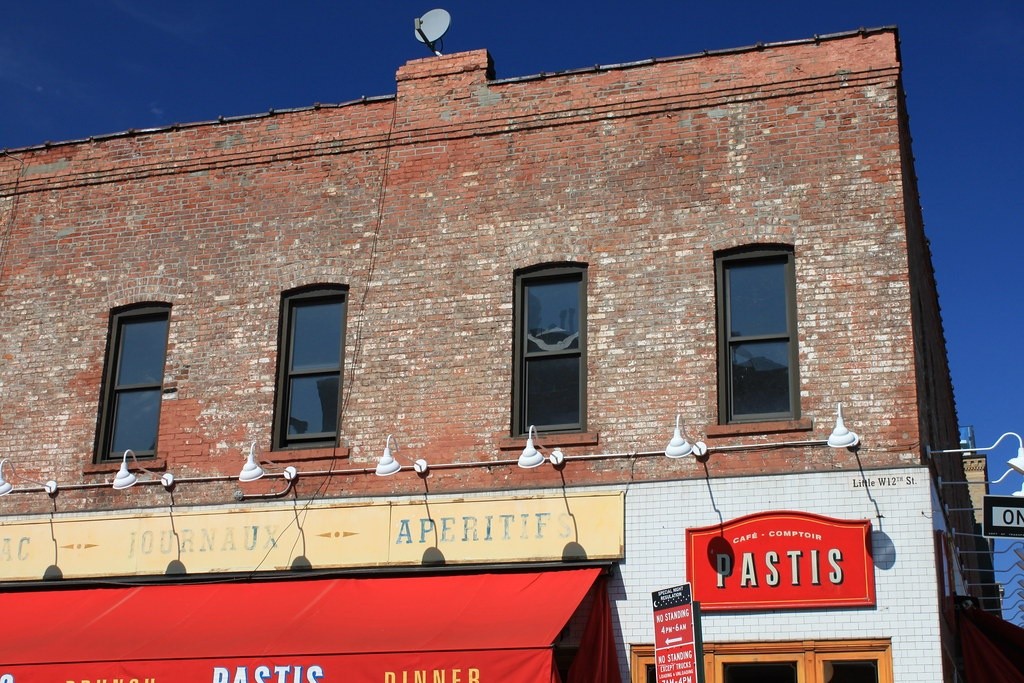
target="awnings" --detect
[0,568,604,683]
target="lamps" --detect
[664,414,707,459]
[827,402,860,448]
[375,434,427,476]
[238,442,297,482]
[0,458,58,497]
[926,432,1024,627]
[517,425,564,469]
[112,449,174,489]
